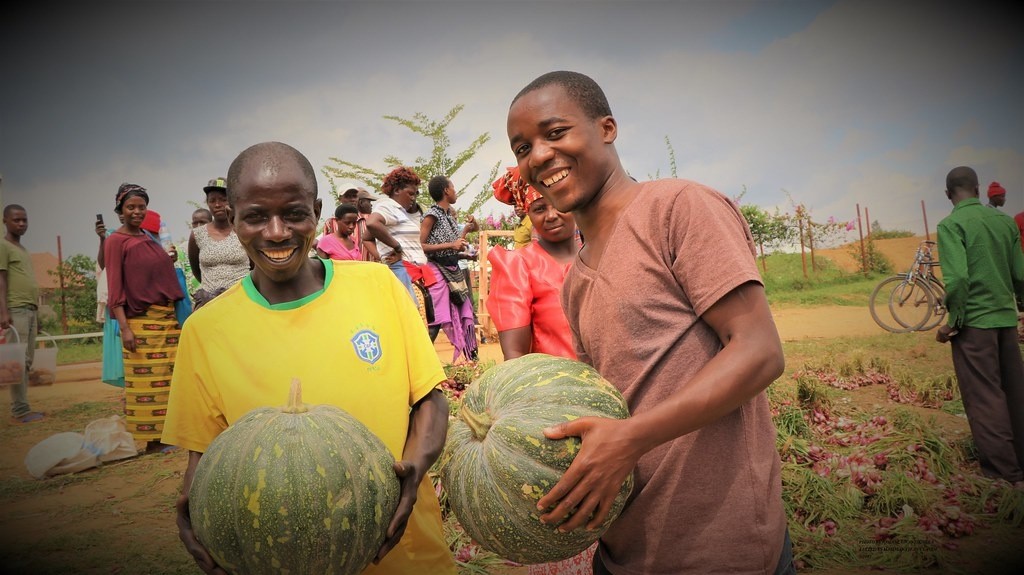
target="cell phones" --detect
[96,214,103,225]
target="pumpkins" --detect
[440,353,634,563]
[188,379,400,575]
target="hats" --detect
[358,190,378,201]
[338,183,358,199]
[203,177,228,194]
[987,182,1006,199]
[138,211,161,233]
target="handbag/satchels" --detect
[173,267,192,326]
[436,263,468,306]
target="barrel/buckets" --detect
[28,331,58,385]
[0,324,27,385]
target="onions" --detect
[441,362,999,569]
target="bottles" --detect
[159,223,175,256]
[465,242,475,255]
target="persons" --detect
[487,166,582,360]
[96,211,192,388]
[936,166,1024,484]
[312,186,376,261]
[508,72,794,575]
[420,175,478,359]
[104,184,182,452]
[163,143,457,575]
[0,205,50,422]
[189,178,252,306]
[514,205,533,249]
[192,209,211,228]
[363,167,439,347]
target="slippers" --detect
[8,412,46,424]
[146,445,177,454]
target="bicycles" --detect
[870,241,948,333]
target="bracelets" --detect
[394,243,401,250]
